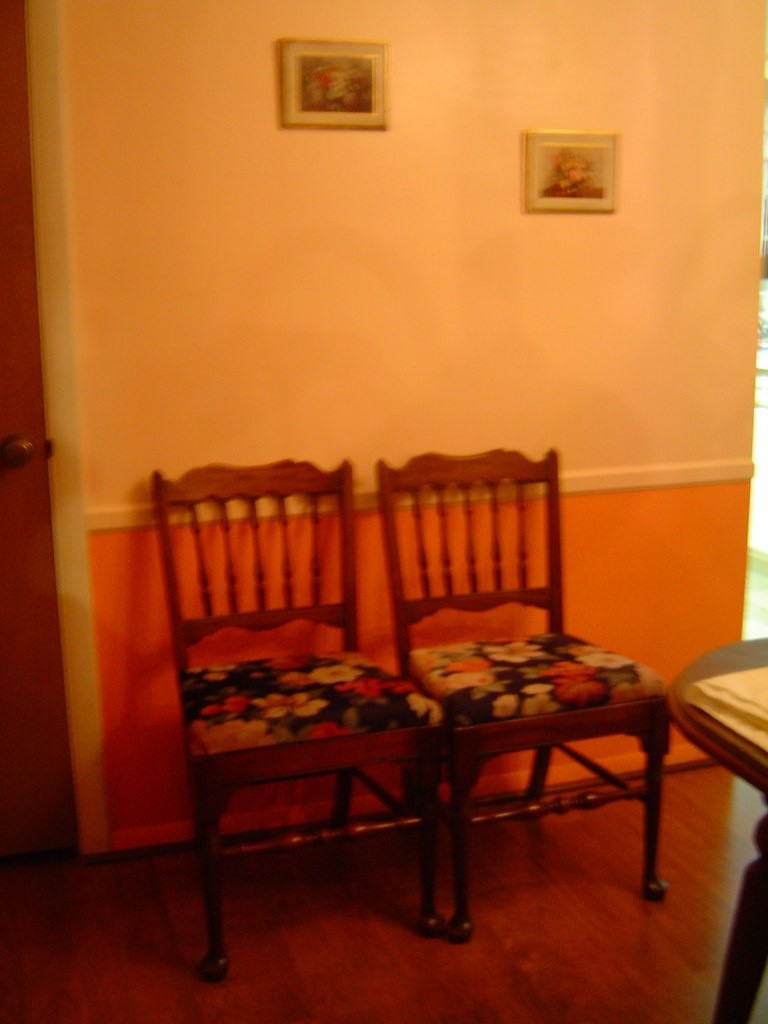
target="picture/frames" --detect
[523,131,619,214]
[277,39,387,131]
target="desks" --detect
[666,639,768,1024]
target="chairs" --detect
[377,446,671,945]
[149,460,451,982]
